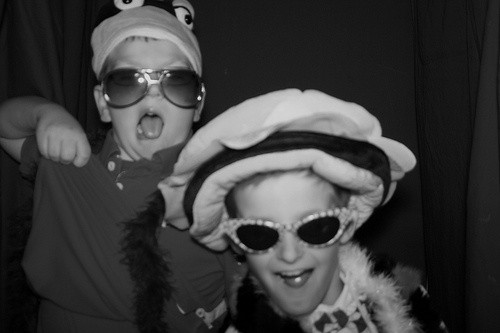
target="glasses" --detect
[101,67,205,110]
[222,206,354,254]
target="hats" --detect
[86,0,202,83]
[154,88,418,255]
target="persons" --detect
[0,0,245,333]
[159,88,449,332]
